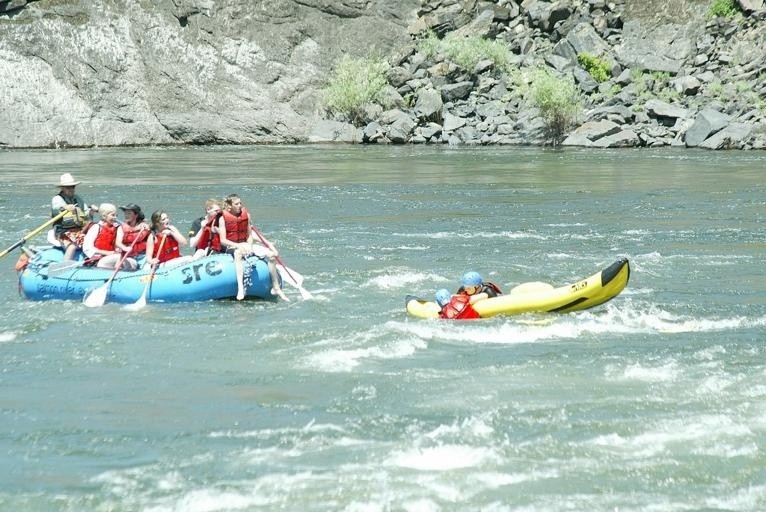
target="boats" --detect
[406,258,632,324]
[16,247,281,308]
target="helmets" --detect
[461,271,481,289]
[434,288,451,308]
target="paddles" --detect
[251,225,313,300]
[135,234,167,307]
[85,227,145,308]
[48,255,101,276]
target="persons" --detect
[188,198,220,253]
[144,208,187,267]
[207,196,228,252]
[115,203,151,274]
[456,272,502,297]
[82,201,123,270]
[218,193,291,302]
[433,288,489,320]
[50,172,92,261]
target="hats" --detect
[119,204,141,215]
[54,173,82,188]
